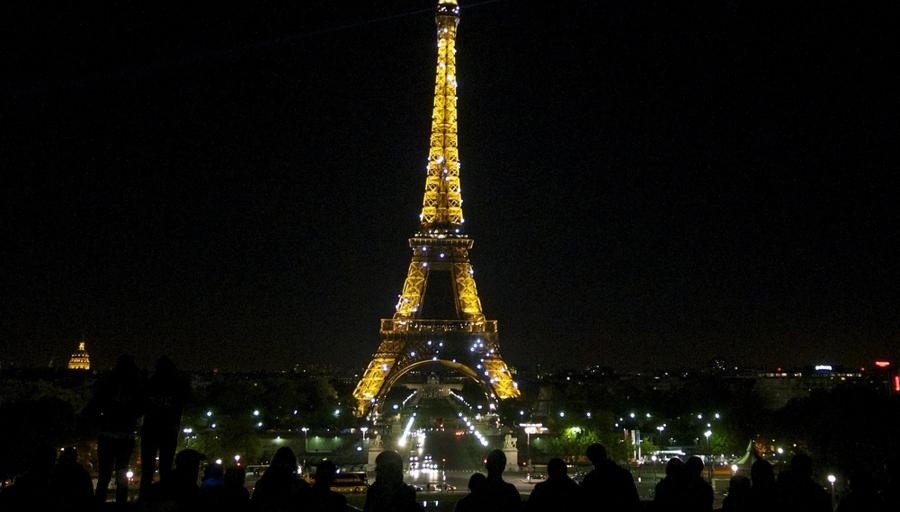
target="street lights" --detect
[183,427,193,437]
[301,427,310,451]
[656,425,665,437]
[360,427,369,440]
[704,430,713,479]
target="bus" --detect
[304,471,371,494]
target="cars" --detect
[405,418,468,491]
[704,454,728,466]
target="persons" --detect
[2,354,900,511]
